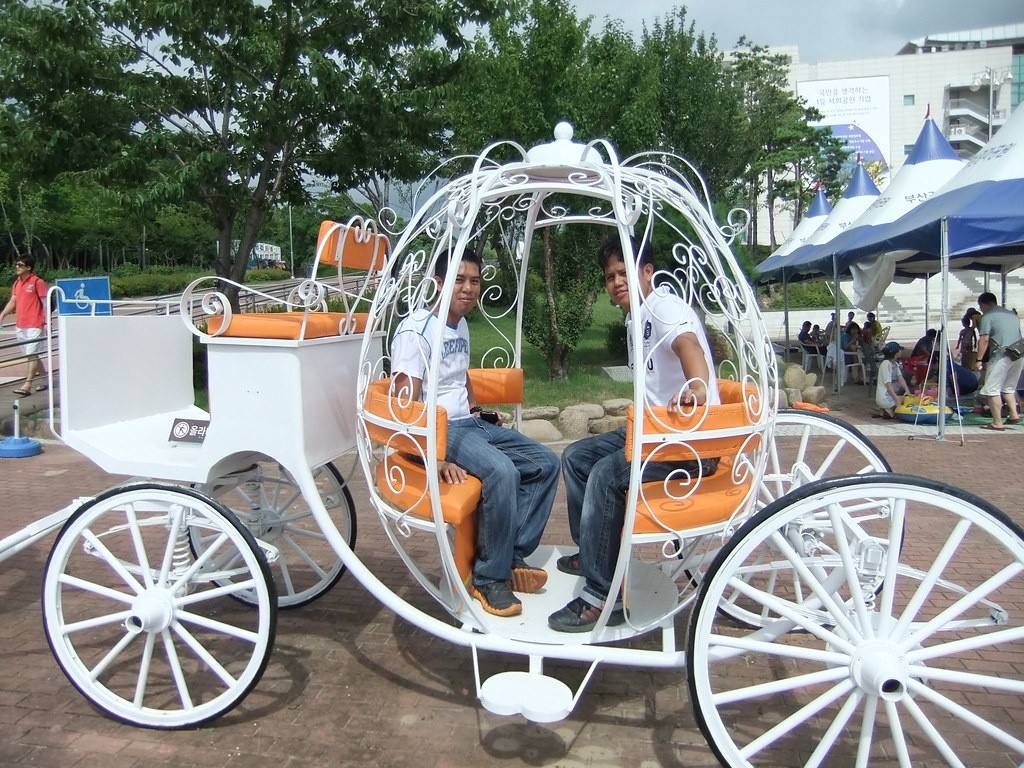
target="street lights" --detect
[969,65,1013,141]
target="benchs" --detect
[365,368,525,526]
[208,221,387,345]
[626,377,767,545]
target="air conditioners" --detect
[956,127,965,134]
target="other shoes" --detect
[855,381,863,384]
[872,413,884,418]
[883,414,897,419]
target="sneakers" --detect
[548,596,630,633]
[557,553,583,576]
[469,581,523,617]
[506,558,548,593]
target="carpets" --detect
[921,390,1024,426]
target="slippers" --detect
[35,385,48,391]
[1003,418,1020,424]
[12,389,31,395]
[980,424,1005,431]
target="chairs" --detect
[797,325,891,388]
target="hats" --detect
[885,342,904,354]
[964,308,981,318]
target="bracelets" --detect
[975,358,982,362]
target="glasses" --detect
[15,263,27,267]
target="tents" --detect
[783,154,930,362]
[753,184,833,303]
[787,103,990,396]
[838,101,1024,447]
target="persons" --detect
[956,318,977,368]
[975,292,1024,430]
[0,254,49,396]
[388,249,560,617]
[799,311,882,384]
[546,235,722,633]
[965,308,982,334]
[876,328,978,420]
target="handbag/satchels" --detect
[1005,339,1024,361]
